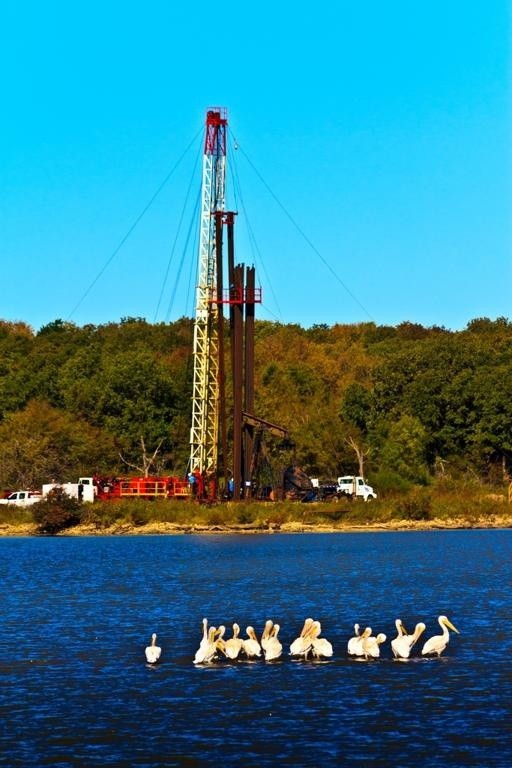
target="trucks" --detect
[326,474,378,502]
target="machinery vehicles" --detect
[78,107,227,501]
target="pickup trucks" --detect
[0,490,42,510]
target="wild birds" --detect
[391,618,426,658]
[288,618,334,661]
[347,623,387,658]
[191,618,283,666]
[144,633,162,664]
[421,615,460,657]
